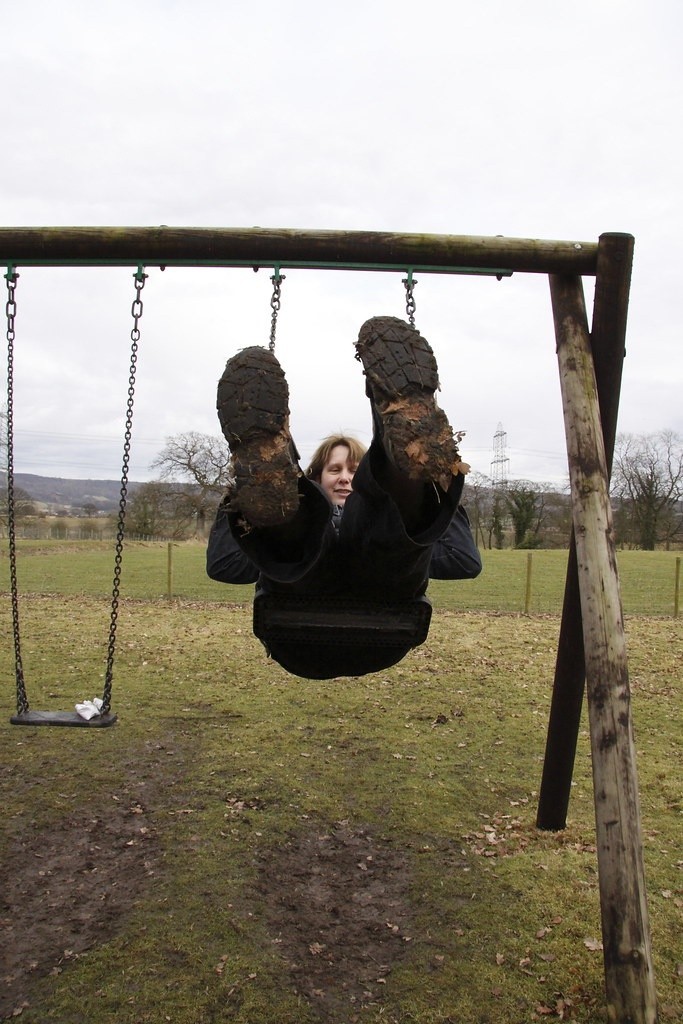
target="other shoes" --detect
[216,345,299,523]
[358,316,461,485]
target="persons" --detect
[205,315,482,681]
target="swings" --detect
[251,279,434,651]
[6,278,144,728]
